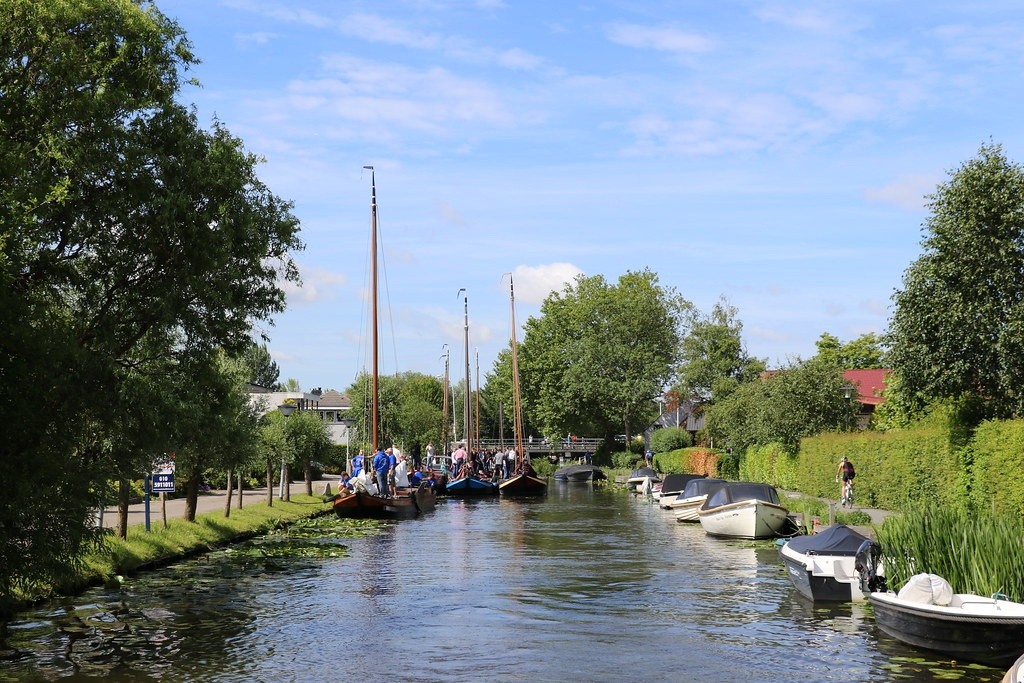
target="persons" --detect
[338,441,515,499]
[836,457,855,505]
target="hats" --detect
[393,444,399,448]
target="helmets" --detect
[841,457,847,461]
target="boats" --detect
[868,572,1023,683]
[624,468,662,495]
[651,473,730,523]
[552,470,593,482]
[697,482,791,540]
[780,523,879,602]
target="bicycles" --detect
[834,476,854,509]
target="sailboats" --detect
[497,271,550,497]
[333,163,446,520]
[446,288,497,498]
